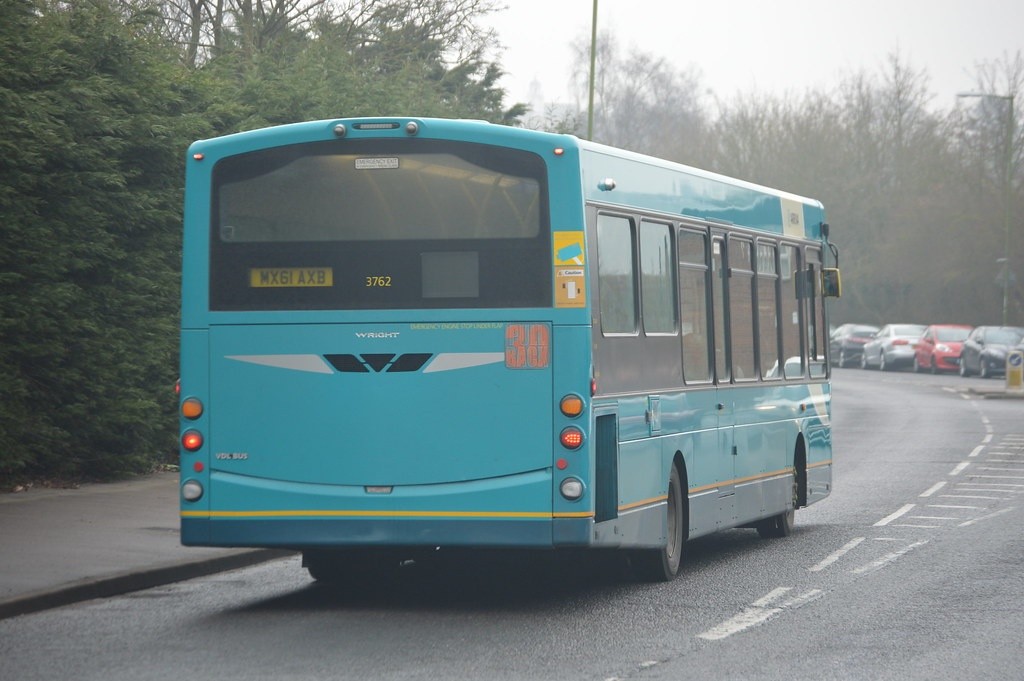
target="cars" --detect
[860,323,928,372]
[827,323,882,369]
[957,325,1024,378]
[911,323,974,374]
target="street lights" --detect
[956,90,1015,323]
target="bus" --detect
[176,112,842,582]
